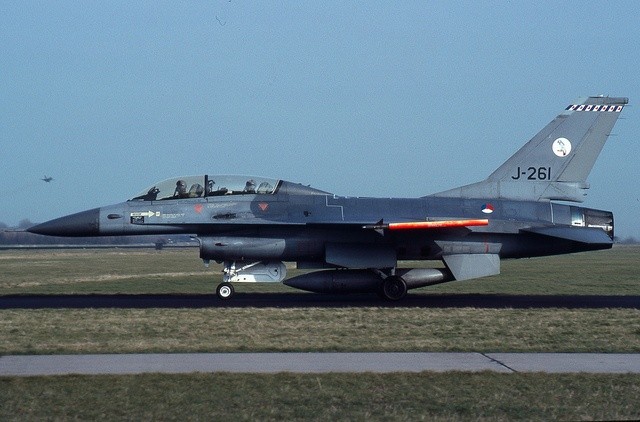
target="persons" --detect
[244,178,258,193]
[175,181,188,200]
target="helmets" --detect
[177,180,187,192]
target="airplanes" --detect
[4,97,629,299]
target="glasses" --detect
[246,181,254,186]
[176,181,184,186]
